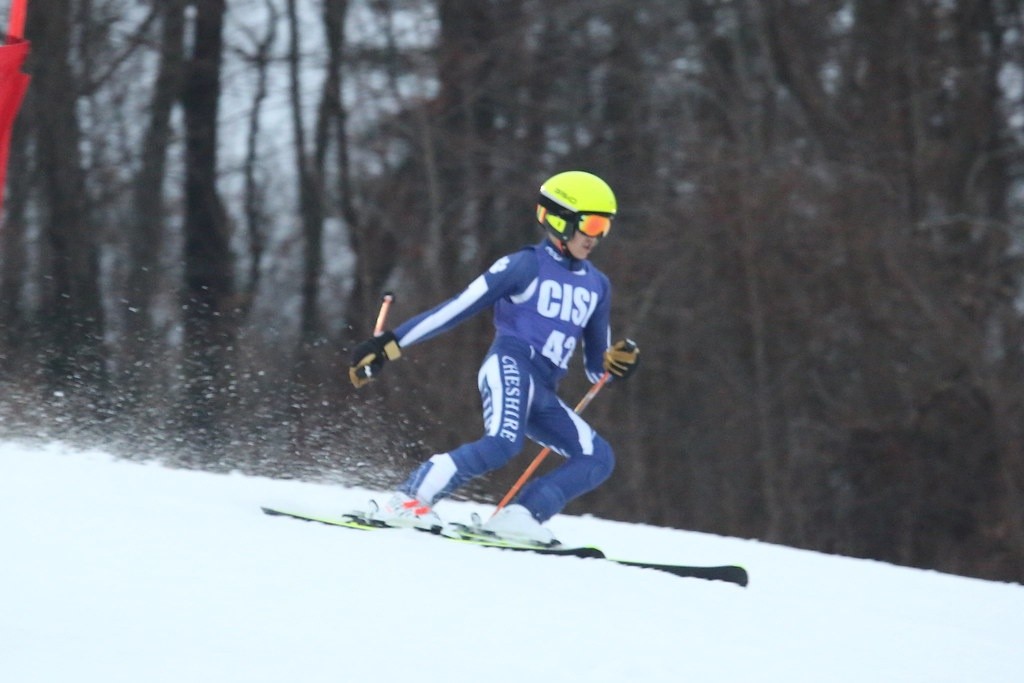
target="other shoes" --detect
[482,508,561,548]
[371,490,442,531]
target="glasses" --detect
[575,212,610,237]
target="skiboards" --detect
[260,495,748,589]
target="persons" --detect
[348,171,641,548]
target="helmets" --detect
[535,172,617,241]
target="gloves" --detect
[349,331,401,388]
[603,338,640,380]
